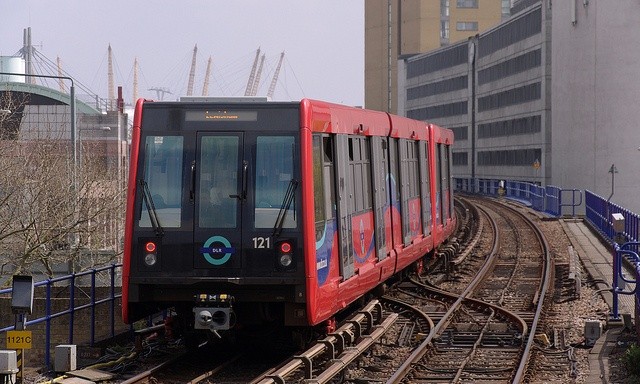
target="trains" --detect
[123,97,456,351]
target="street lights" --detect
[1,71,76,248]
[78,127,112,202]
